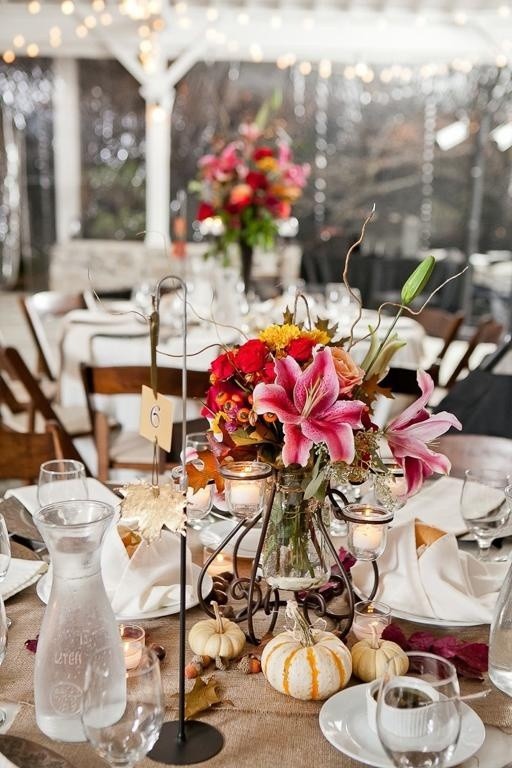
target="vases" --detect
[262,470,331,589]
[233,232,262,303]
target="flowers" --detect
[182,204,470,579]
[191,88,311,266]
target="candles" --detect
[230,465,262,506]
[353,509,382,551]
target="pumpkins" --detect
[351,624,409,682]
[259,599,353,700]
[188,600,244,657]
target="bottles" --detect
[32,501,127,742]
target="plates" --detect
[197,518,262,561]
[319,684,485,766]
[35,561,215,621]
[349,558,502,629]
[386,476,504,535]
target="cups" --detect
[367,677,438,736]
[188,433,209,451]
[352,600,392,641]
[117,624,145,669]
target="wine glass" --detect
[459,469,511,561]
[38,460,88,508]
[79,643,166,767]
[135,266,358,326]
[0,596,6,725]
[1,513,11,627]
[375,649,462,767]
